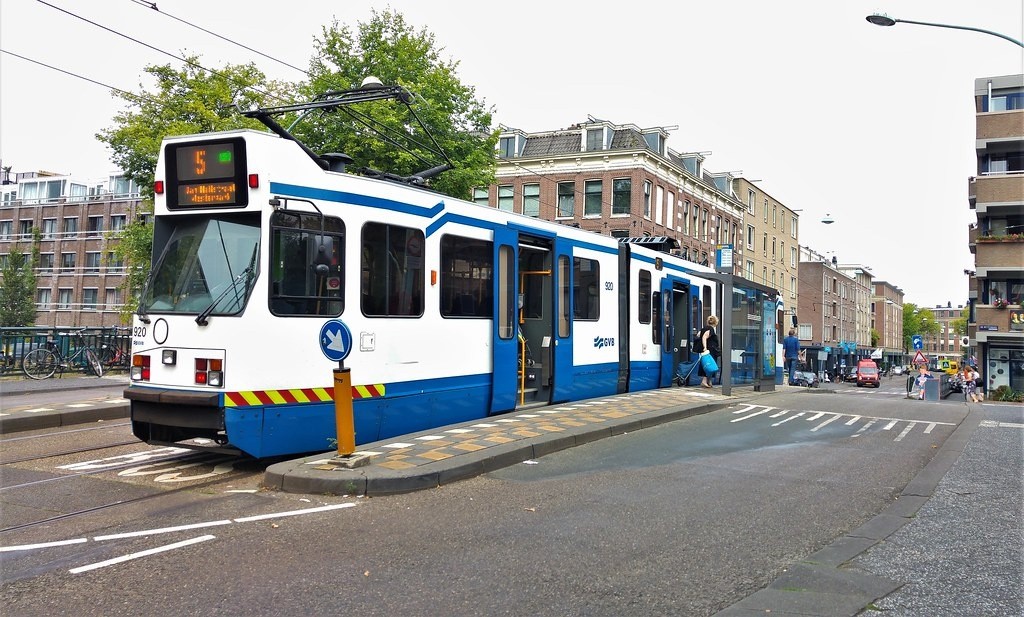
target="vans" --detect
[856,359,880,388]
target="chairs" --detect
[1012,292,1020,305]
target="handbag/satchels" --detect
[975,375,983,387]
[700,352,719,375]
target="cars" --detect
[9,343,44,361]
[844,366,858,383]
[786,370,820,388]
[817,371,826,382]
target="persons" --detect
[1011,311,1024,324]
[963,365,983,403]
[915,366,935,400]
[781,330,806,386]
[697,315,719,388]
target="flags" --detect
[836,339,857,354]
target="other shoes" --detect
[701,383,711,388]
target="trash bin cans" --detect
[907,376,916,392]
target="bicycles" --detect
[22,326,103,380]
[87,324,131,375]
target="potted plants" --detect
[977,235,998,242]
[1018,234,1024,242]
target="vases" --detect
[1001,239,1016,243]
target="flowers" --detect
[1009,233,1019,239]
[993,298,1008,307]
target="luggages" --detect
[674,356,701,387]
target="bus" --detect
[125,85,785,460]
[945,361,959,375]
